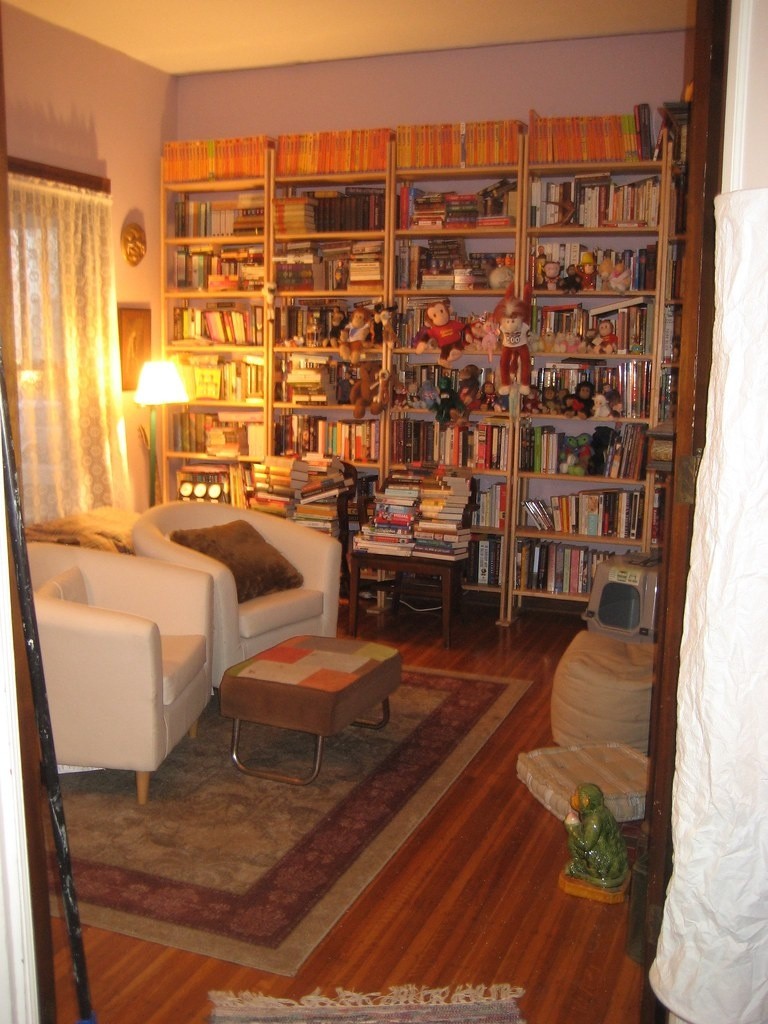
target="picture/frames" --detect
[117,306,154,397]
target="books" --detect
[528,243,657,290]
[164,135,278,184]
[170,301,265,508]
[394,119,528,170]
[174,193,264,238]
[276,128,396,175]
[351,461,504,588]
[396,238,514,530]
[659,107,690,302]
[273,187,386,234]
[400,178,517,230]
[528,105,667,164]
[171,244,264,291]
[247,457,349,539]
[516,297,654,593]
[274,239,385,461]
[652,306,682,546]
[528,171,660,228]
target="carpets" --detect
[43,660,536,981]
[204,982,528,1024]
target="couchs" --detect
[129,498,344,694]
[22,538,218,807]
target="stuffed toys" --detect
[322,280,625,477]
[535,252,631,297]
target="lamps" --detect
[130,353,194,510]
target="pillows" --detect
[169,515,304,605]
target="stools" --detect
[219,633,406,787]
[346,546,470,653]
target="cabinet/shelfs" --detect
[156,99,695,632]
